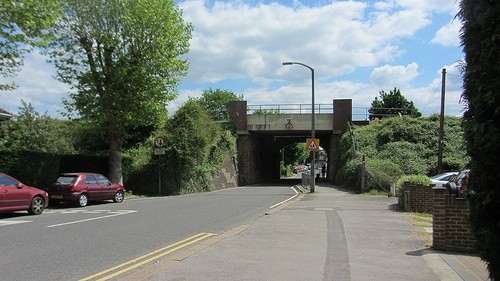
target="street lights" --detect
[282,60,317,194]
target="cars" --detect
[0,171,50,216]
[292,163,312,175]
[48,172,125,207]
